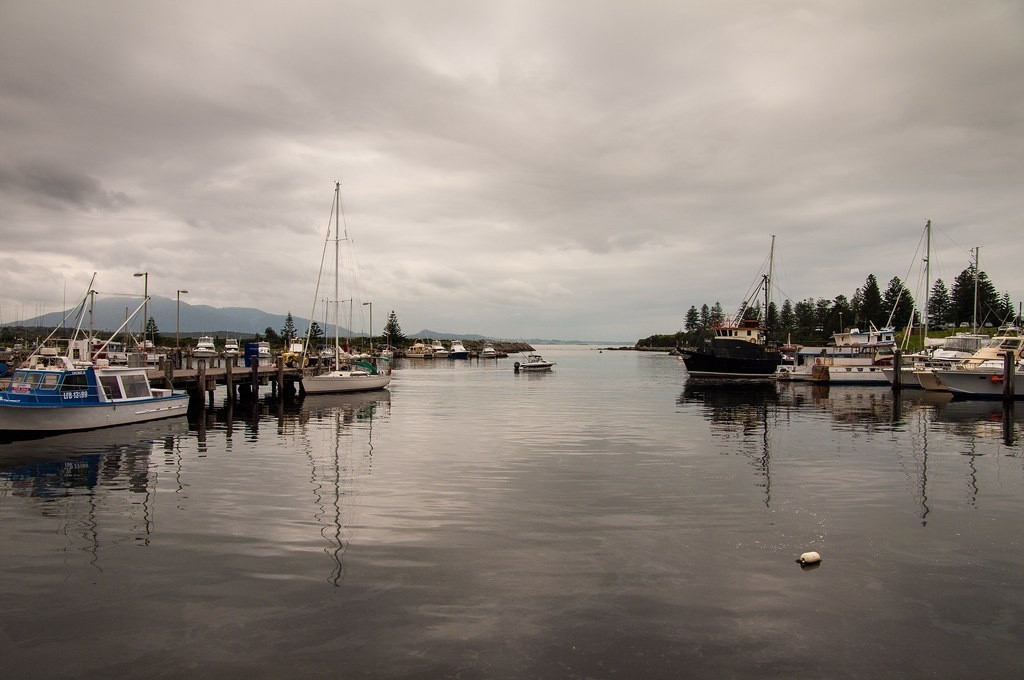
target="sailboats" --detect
[677,273,786,378]
[879,218,1023,403]
[289,180,393,394]
[675,378,781,475]
[288,389,394,591]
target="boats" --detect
[931,397,1024,425]
[0,361,190,433]
[20,334,155,377]
[514,353,556,373]
[190,335,513,359]
[0,415,191,572]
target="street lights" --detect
[176,289,189,352]
[133,271,148,353]
[360,301,373,356]
